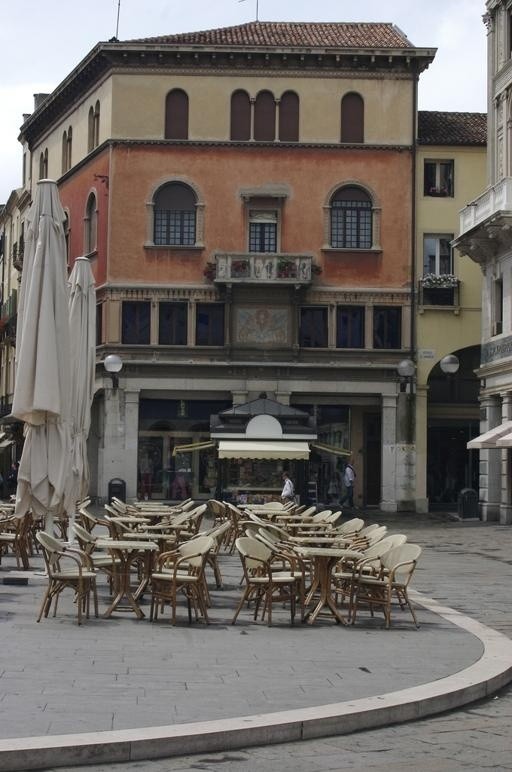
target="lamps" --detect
[439,354,480,382]
[101,354,123,388]
[397,359,416,395]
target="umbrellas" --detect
[11,178,96,543]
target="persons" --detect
[139,451,153,500]
[0,462,20,500]
[280,470,294,504]
[336,460,356,507]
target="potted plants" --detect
[277,256,292,278]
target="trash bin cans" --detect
[108,478,126,506]
[457,488,480,519]
